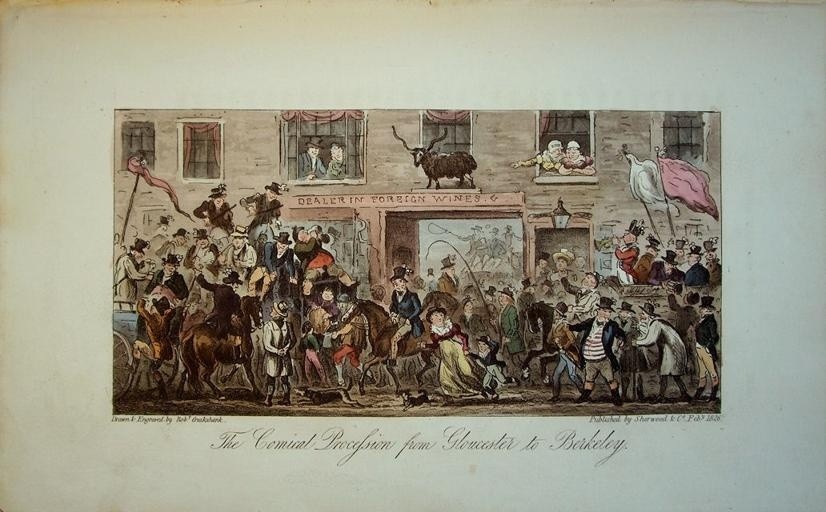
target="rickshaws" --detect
[113,301,181,404]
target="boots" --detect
[637,377,646,401]
[706,383,719,403]
[576,389,592,403]
[278,383,291,406]
[620,377,634,402]
[257,383,275,406]
[611,387,623,406]
[684,386,705,402]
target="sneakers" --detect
[549,396,559,401]
[387,359,396,368]
[648,394,669,403]
[491,394,499,401]
[511,376,519,383]
[673,392,692,403]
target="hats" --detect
[265,182,282,195]
[292,225,304,241]
[331,142,344,152]
[625,219,685,265]
[165,255,178,264]
[337,293,351,303]
[539,252,550,262]
[441,256,456,269]
[194,228,209,239]
[585,271,601,288]
[689,246,703,256]
[687,291,700,305]
[173,229,187,240]
[208,188,227,199]
[152,296,170,316]
[274,232,293,245]
[273,300,289,318]
[553,302,568,315]
[130,239,149,255]
[699,296,715,309]
[567,140,581,150]
[476,335,490,346]
[304,135,325,149]
[390,267,408,282]
[553,252,573,266]
[541,280,554,288]
[426,306,447,323]
[548,139,564,153]
[703,241,717,253]
[223,271,243,285]
[596,297,662,318]
[230,225,248,237]
[462,278,531,310]
